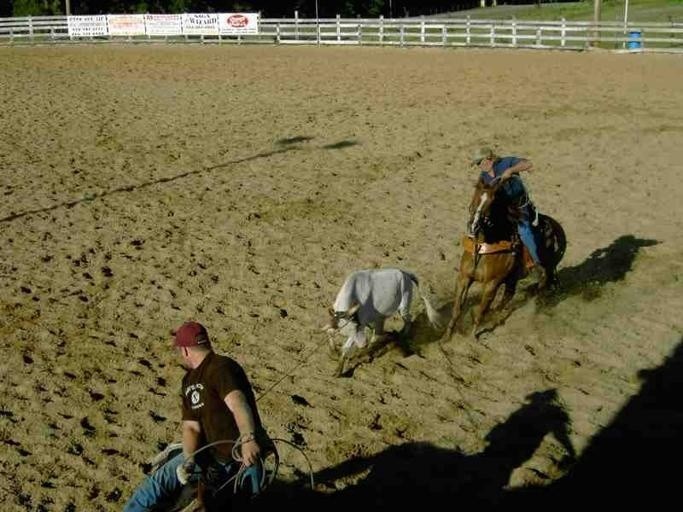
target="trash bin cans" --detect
[630,32,640,48]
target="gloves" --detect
[176,461,196,486]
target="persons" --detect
[466,147,550,290]
[124,320,268,512]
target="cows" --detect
[319,267,444,380]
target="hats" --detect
[172,320,210,348]
[469,144,497,168]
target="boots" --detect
[534,261,554,290]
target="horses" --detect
[173,477,251,512]
[441,175,567,343]
